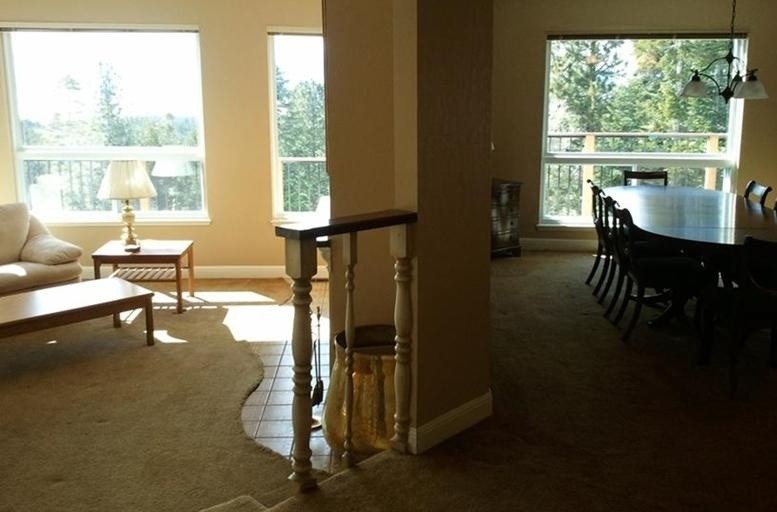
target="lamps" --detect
[677,1,769,105]
[97,159,157,250]
[151,155,192,210]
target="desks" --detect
[320,321,404,467]
[91,241,193,313]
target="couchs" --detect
[0,204,83,292]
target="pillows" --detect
[17,232,83,266]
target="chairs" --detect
[624,170,670,186]
[585,180,636,296]
[695,235,776,400]
[613,209,697,343]
[598,198,669,317]
[742,180,771,208]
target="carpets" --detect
[0,314,334,512]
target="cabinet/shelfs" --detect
[488,180,522,259]
